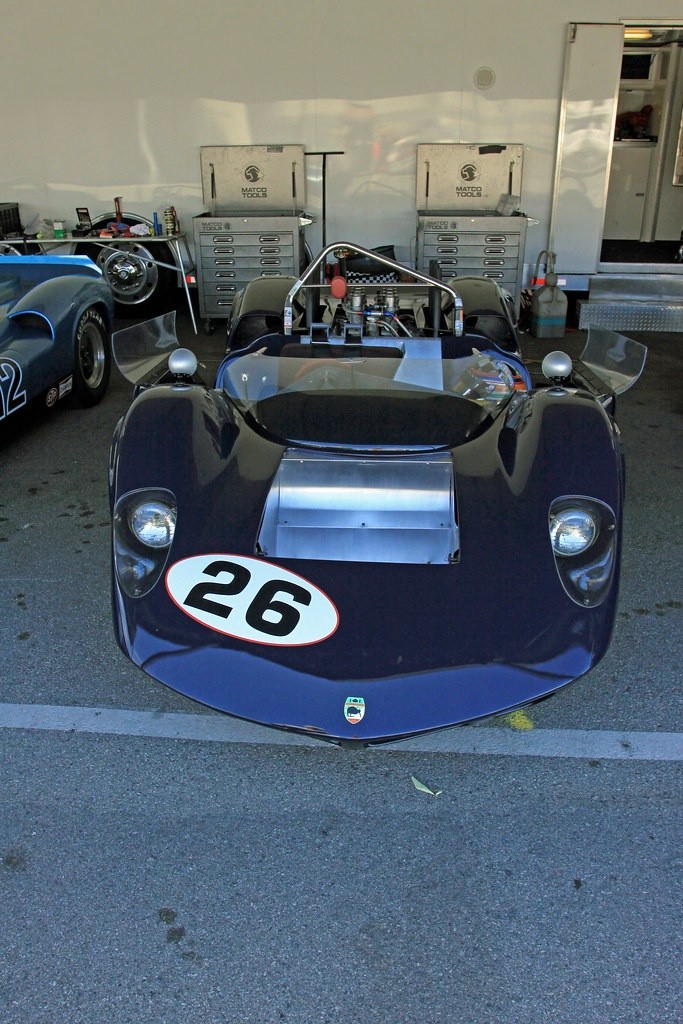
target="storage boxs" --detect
[0,201,22,236]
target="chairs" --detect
[223,311,282,350]
[462,307,524,354]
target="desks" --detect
[0,226,199,337]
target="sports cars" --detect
[0,229,117,420]
[105,241,648,751]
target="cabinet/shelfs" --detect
[411,208,527,324]
[196,210,310,319]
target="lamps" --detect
[624,32,665,41]
